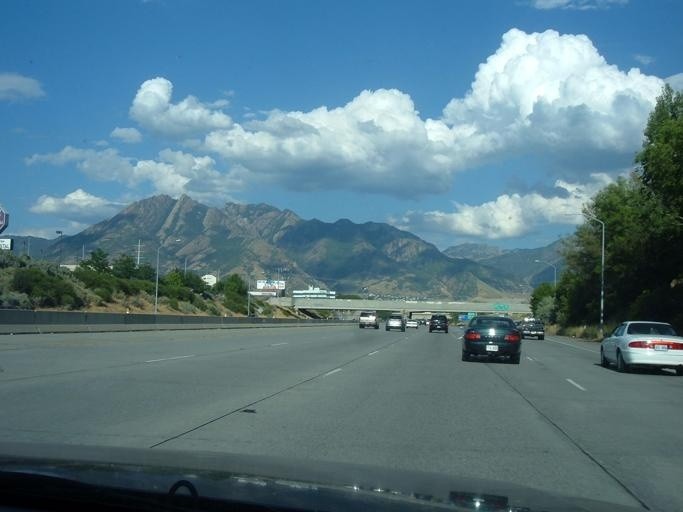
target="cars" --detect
[601,321,683,376]
[428,315,450,333]
[462,317,544,364]
[386,311,429,332]
[359,311,379,329]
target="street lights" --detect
[248,272,265,317]
[565,212,605,338]
[520,285,533,291]
[155,239,181,313]
[535,260,556,287]
[56,231,62,255]
